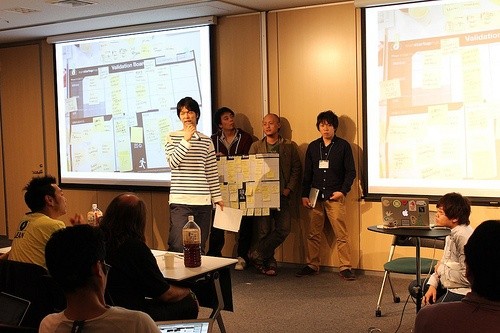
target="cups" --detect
[163,253,175,267]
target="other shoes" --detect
[235,256,246,271]
[338,268,356,280]
[255,257,278,277]
[296,265,315,277]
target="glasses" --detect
[97,262,112,272]
[436,207,447,216]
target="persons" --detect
[415,220,500,333]
[39,223,160,333]
[296,111,356,280]
[8,176,66,269]
[408,193,473,306]
[165,97,224,256]
[211,107,253,270]
[249,114,300,274]
[99,194,199,321]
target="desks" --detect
[151,249,238,333]
[368,225,451,317]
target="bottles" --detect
[182,216,202,268]
[87,203,103,227]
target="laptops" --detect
[380,198,431,231]
[0,292,31,327]
[154,318,213,333]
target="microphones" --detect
[185,122,202,141]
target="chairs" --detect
[375,211,445,317]
